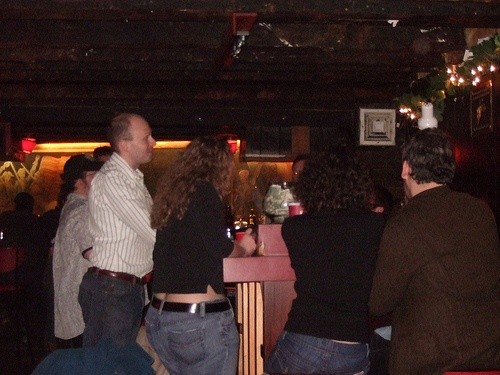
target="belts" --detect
[152,296,231,313]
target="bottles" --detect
[249,209,255,225]
[225,205,234,239]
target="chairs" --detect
[0,244,51,370]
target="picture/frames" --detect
[469,86,494,138]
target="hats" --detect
[64,155,105,181]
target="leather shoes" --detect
[88,266,143,285]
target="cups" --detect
[288,202,304,218]
[235,232,245,240]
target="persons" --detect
[77,113,158,348]
[370,133,499,375]
[1,192,50,328]
[49,152,103,349]
[290,154,308,180]
[143,132,257,375]
[92,145,113,162]
[263,140,390,375]
